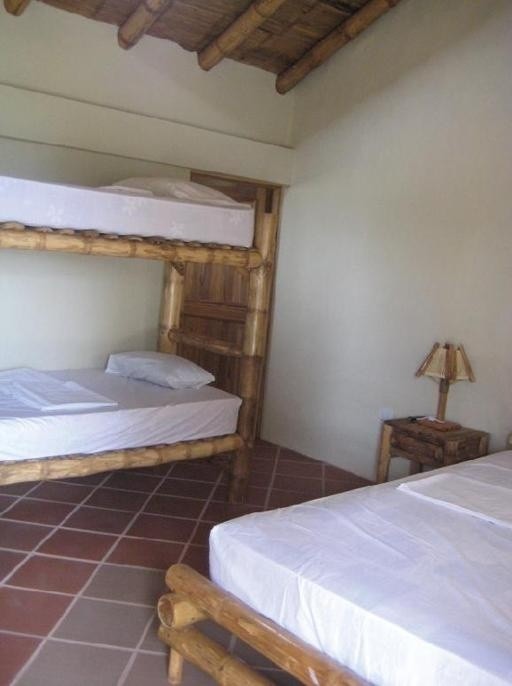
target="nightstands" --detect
[376,415,490,483]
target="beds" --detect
[156,431,511,686]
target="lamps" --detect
[414,342,475,433]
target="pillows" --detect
[105,350,215,390]
[111,177,238,202]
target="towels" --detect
[0,175,279,504]
[0,367,118,412]
[395,470,511,529]
[99,186,153,194]
